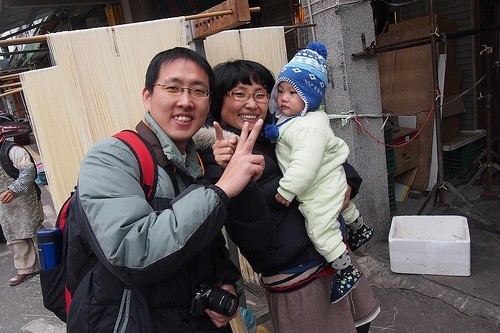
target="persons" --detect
[0,133,44,286]
[66,47,266,333]
[192,59,381,333]
[265,41,374,305]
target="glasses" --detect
[225,90,270,103]
[152,82,213,100]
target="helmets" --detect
[0,126,5,144]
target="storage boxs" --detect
[443,137,486,178]
[384,127,420,215]
[388,215,471,276]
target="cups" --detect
[35,228,62,271]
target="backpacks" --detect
[39,128,158,324]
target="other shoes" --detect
[329,265,365,306]
[348,224,375,252]
[8,273,30,286]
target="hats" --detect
[264,40,329,142]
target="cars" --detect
[0,111,32,145]
